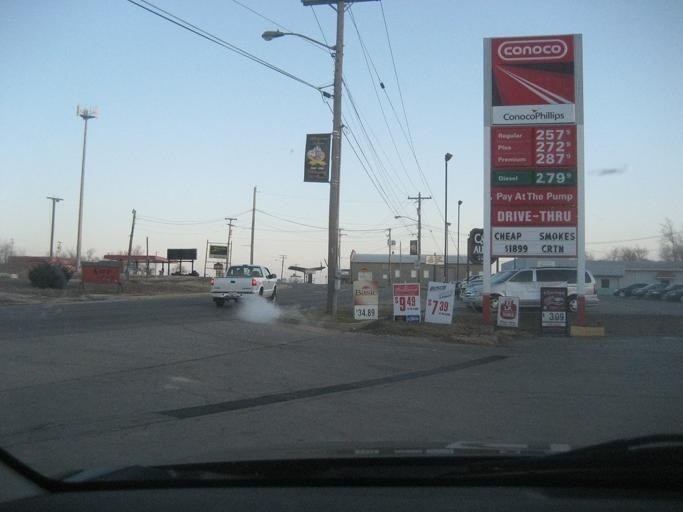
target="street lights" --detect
[455,199,463,285]
[443,151,454,285]
[393,215,422,285]
[71,102,100,273]
[260,29,344,323]
[43,194,64,260]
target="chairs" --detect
[234,270,243,276]
[251,271,260,277]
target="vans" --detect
[454,264,599,316]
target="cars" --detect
[613,281,682,303]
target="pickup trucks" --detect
[209,263,278,308]
